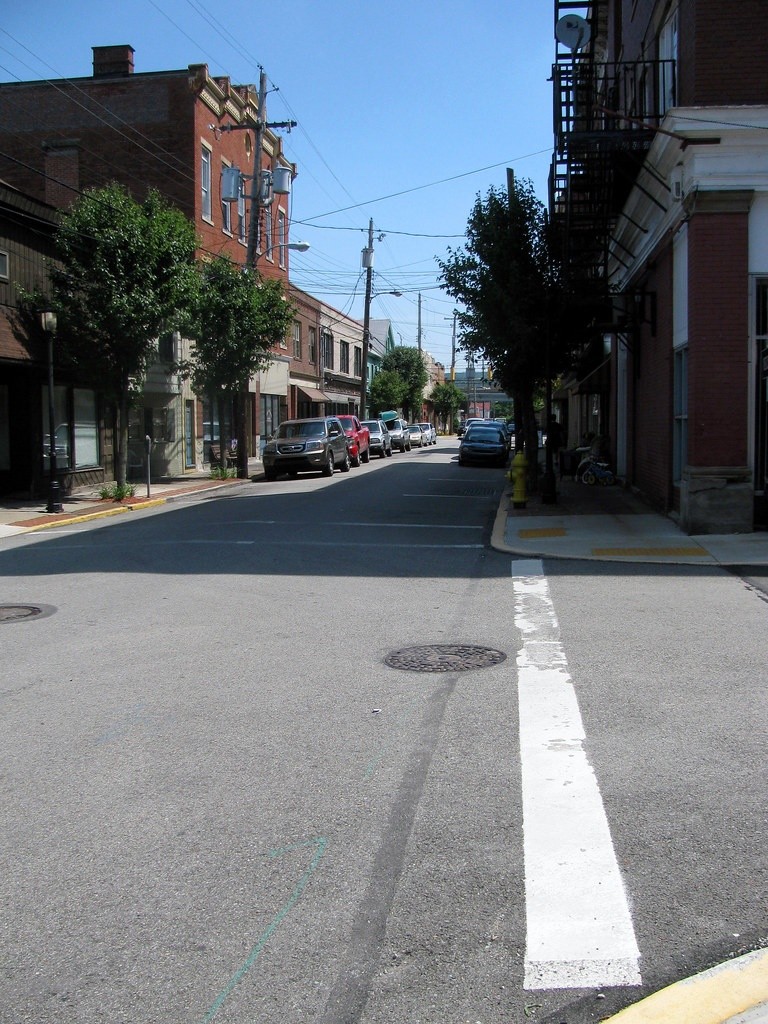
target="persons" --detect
[551,414,563,465]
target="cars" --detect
[407,425,428,447]
[458,417,516,438]
[459,427,510,468]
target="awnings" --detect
[323,392,360,405]
[571,356,611,395]
[297,386,329,402]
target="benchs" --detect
[211,445,237,468]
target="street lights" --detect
[237,242,311,479]
[41,311,65,512]
[360,291,403,421]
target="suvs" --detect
[413,423,436,445]
[384,418,411,452]
[359,418,392,458]
[262,417,351,481]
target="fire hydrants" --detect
[504,449,530,509]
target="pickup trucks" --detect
[327,415,371,467]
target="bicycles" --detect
[581,458,616,487]
